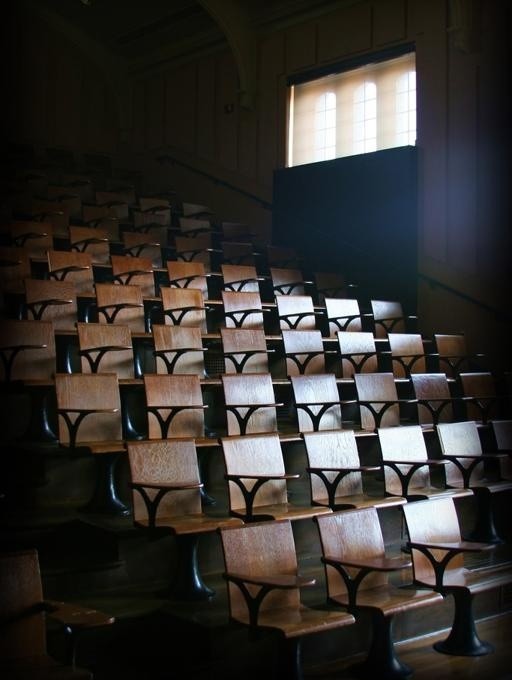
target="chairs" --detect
[2,547,117,679]
[126,419,512,600]
[220,496,511,673]
[0,140,309,265]
[1,265,512,453]
